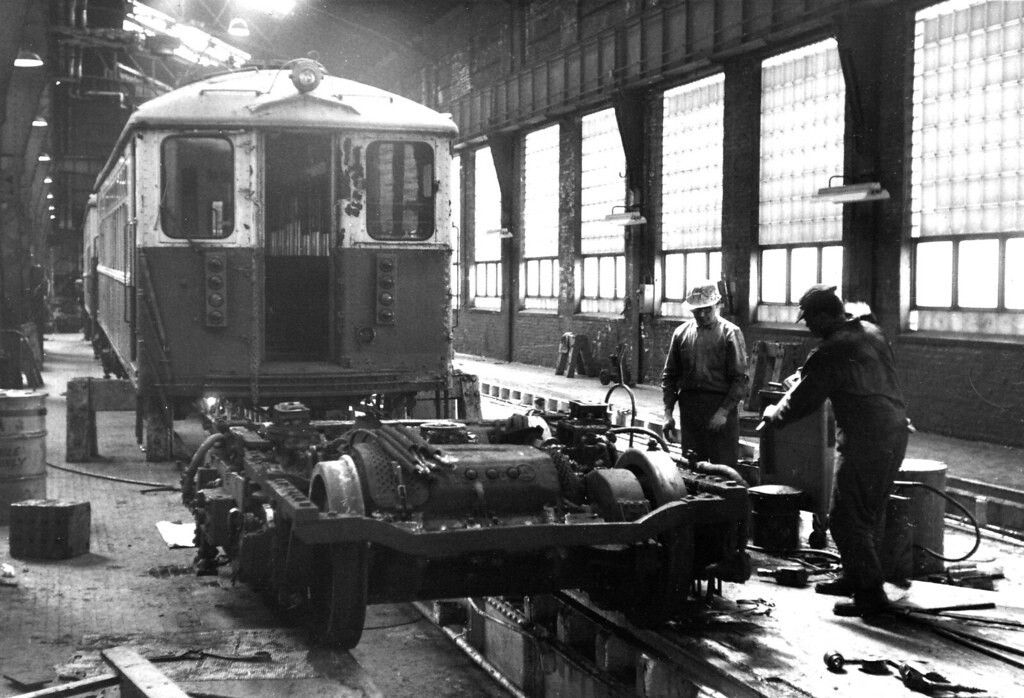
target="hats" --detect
[794,284,837,326]
[684,284,722,309]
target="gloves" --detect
[709,406,728,435]
[661,414,677,444]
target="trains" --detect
[74,59,760,649]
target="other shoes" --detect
[829,592,890,619]
[814,572,884,598]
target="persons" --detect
[660,285,751,472]
[759,281,912,616]
